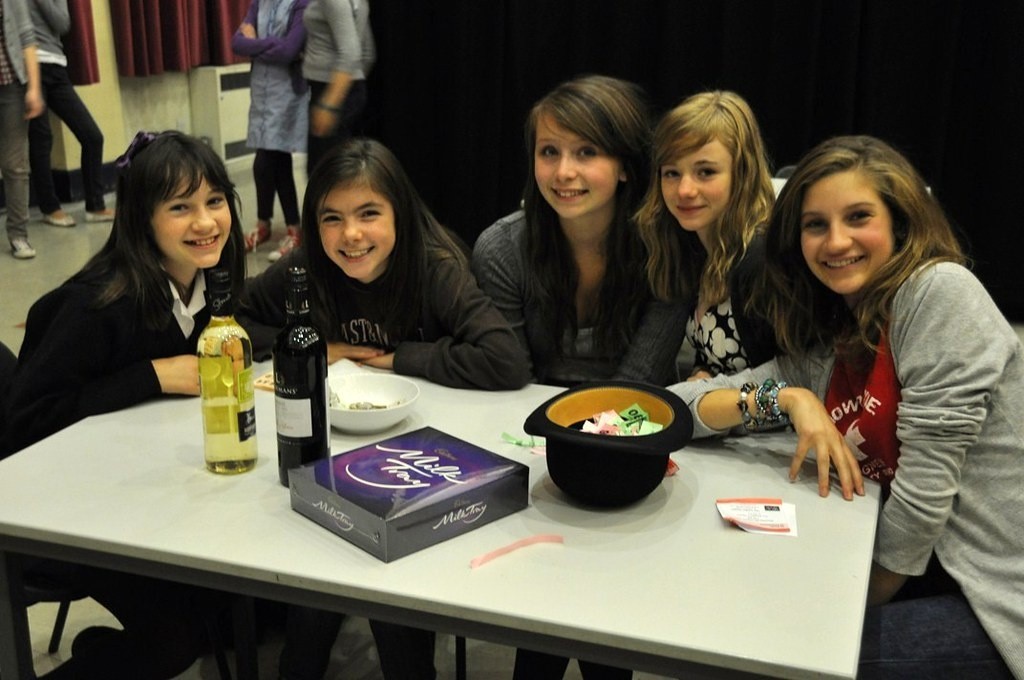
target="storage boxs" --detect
[288,426,528,565]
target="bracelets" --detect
[736,379,791,430]
[316,100,341,113]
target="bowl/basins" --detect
[327,372,420,435]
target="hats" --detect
[524,379,694,508]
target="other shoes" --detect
[43,213,75,227]
[86,212,114,223]
[71,624,121,657]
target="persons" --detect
[0,0,704,679]
[659,136,1023,679]
[635,89,780,379]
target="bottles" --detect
[197,268,258,476]
[273,266,331,488]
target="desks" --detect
[0,357,882,680]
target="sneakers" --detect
[268,231,301,261]
[246,223,271,250]
[10,237,35,260]
